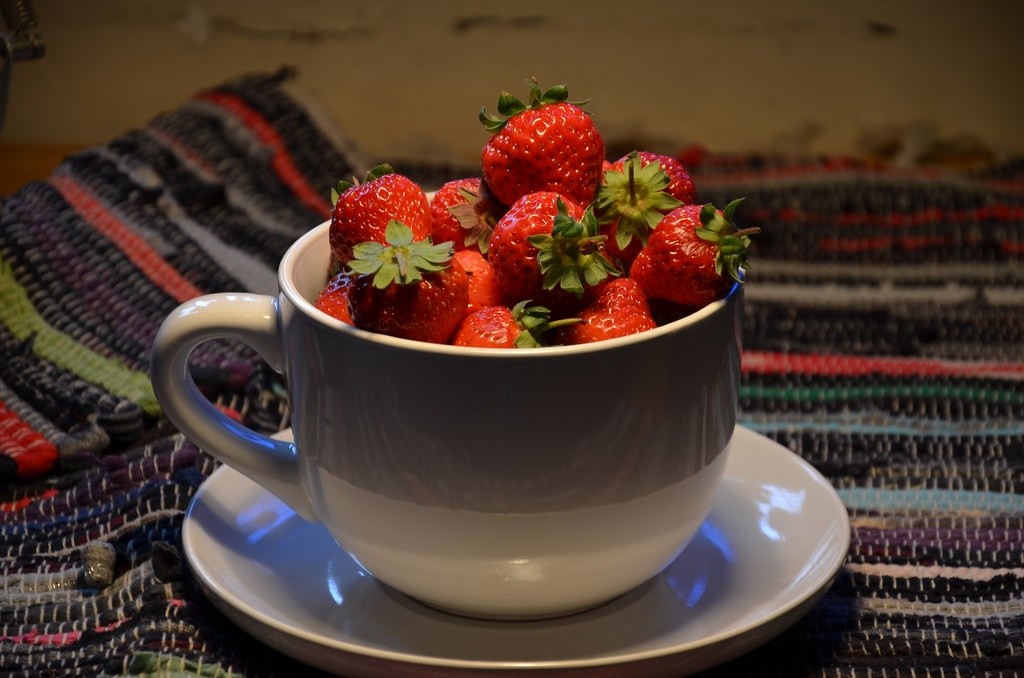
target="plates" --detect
[181,425,851,678]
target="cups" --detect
[147,190,748,622]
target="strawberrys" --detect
[317,80,762,350]
